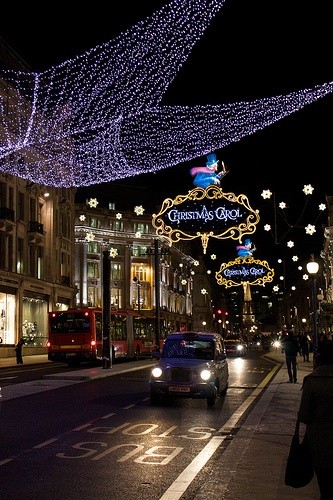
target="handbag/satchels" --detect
[285,418,314,488]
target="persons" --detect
[244,330,333,500]
[14,337,24,364]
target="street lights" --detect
[306,253,319,351]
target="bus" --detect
[46,306,156,365]
[134,307,194,358]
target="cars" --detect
[222,333,282,357]
[148,332,229,408]
